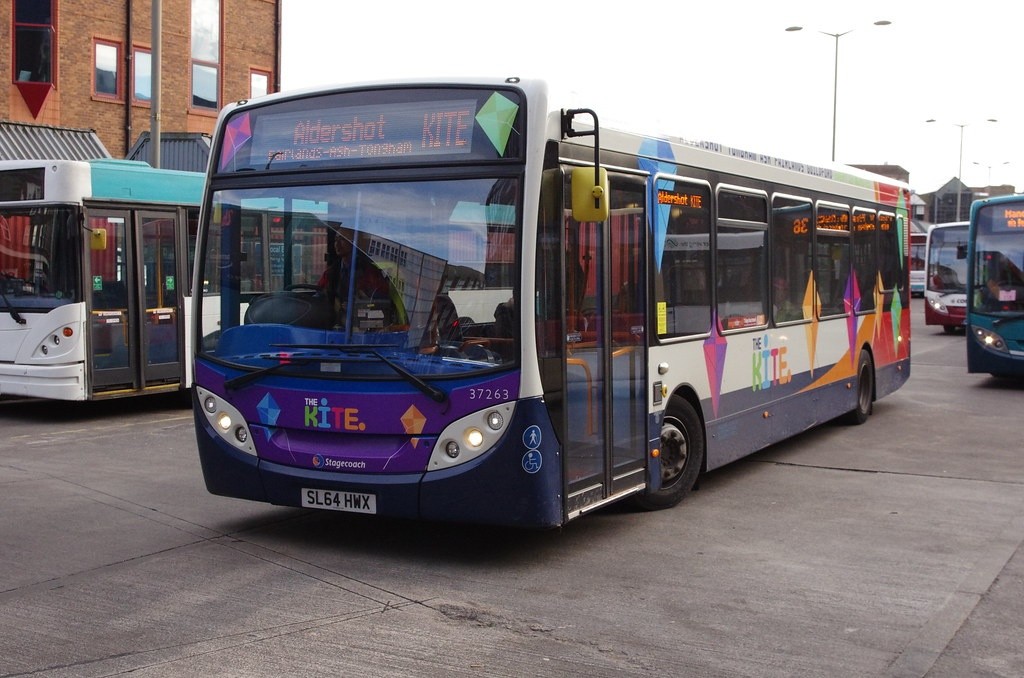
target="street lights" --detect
[927,118,998,224]
[786,21,892,163]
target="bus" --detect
[924,221,971,333]
[966,191,1024,380]
[908,232,930,297]
[190,73,913,533]
[0,158,330,403]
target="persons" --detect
[316,227,389,301]
